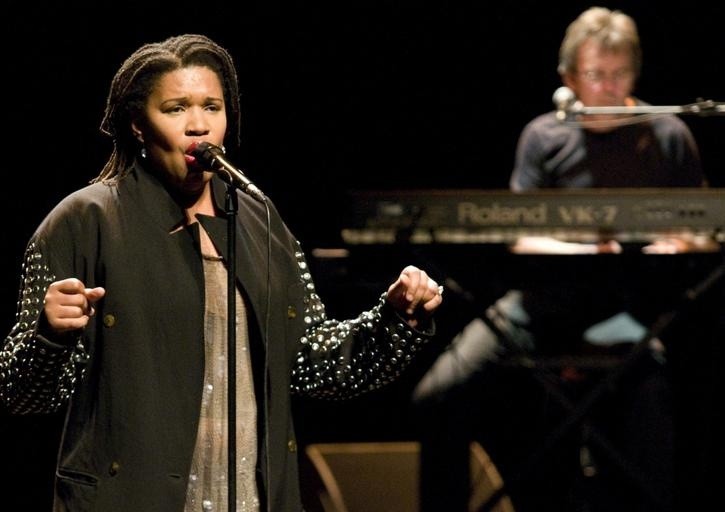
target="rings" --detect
[438,285,444,296]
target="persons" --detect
[0,33,445,506]
[416,3,706,512]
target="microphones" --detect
[552,86,576,123]
[194,142,267,205]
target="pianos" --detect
[342,187,725,245]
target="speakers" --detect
[306,440,512,511]
